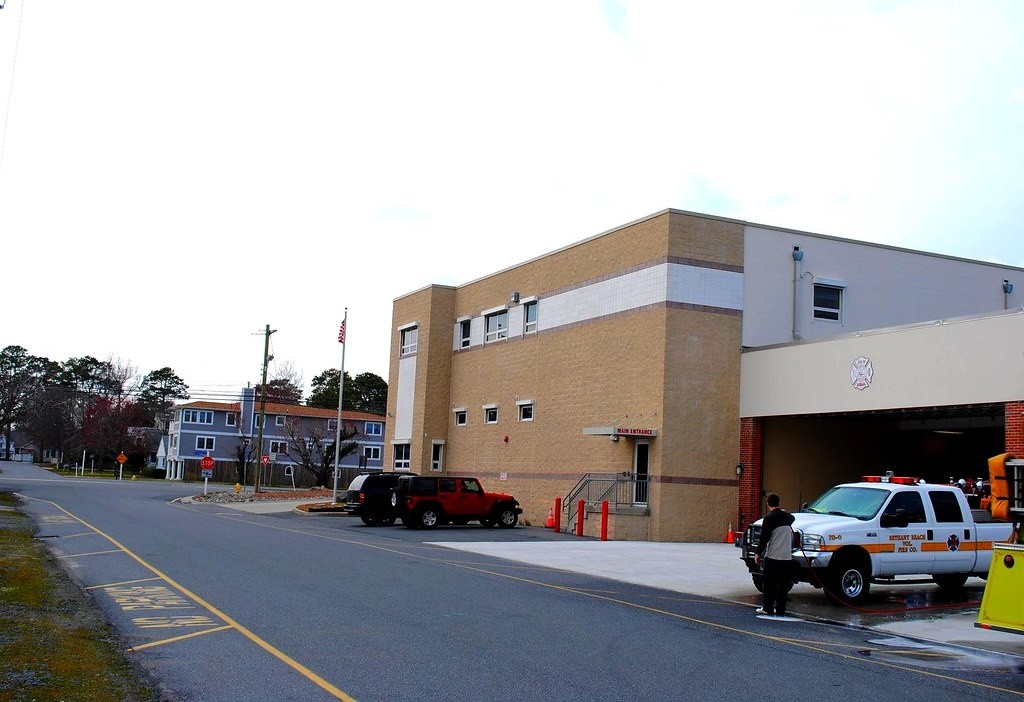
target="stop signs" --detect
[200,456,215,470]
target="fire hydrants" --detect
[235,483,241,493]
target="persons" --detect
[754,494,795,615]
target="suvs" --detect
[390,473,525,530]
[343,470,419,528]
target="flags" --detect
[338,319,345,343]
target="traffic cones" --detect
[545,508,554,528]
[725,520,737,545]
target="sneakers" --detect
[774,610,779,614]
[756,608,768,614]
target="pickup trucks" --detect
[739,469,1022,606]
